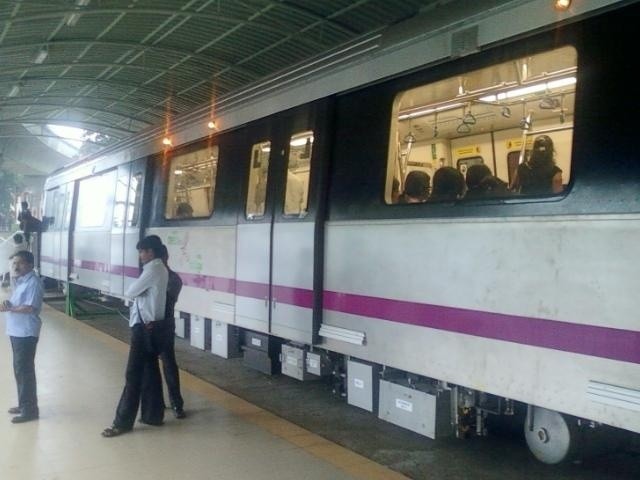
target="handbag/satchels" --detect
[141,319,174,353]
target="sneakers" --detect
[173,405,185,419]
[100,424,125,437]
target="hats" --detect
[136,235,161,249]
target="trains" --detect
[13,1,639,466]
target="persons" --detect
[8,234,25,259]
[101,235,185,437]
[509,134,563,194]
[19,201,31,240]
[0,251,44,424]
[392,164,508,203]
[176,203,193,218]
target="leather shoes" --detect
[8,407,22,413]
[11,415,39,423]
